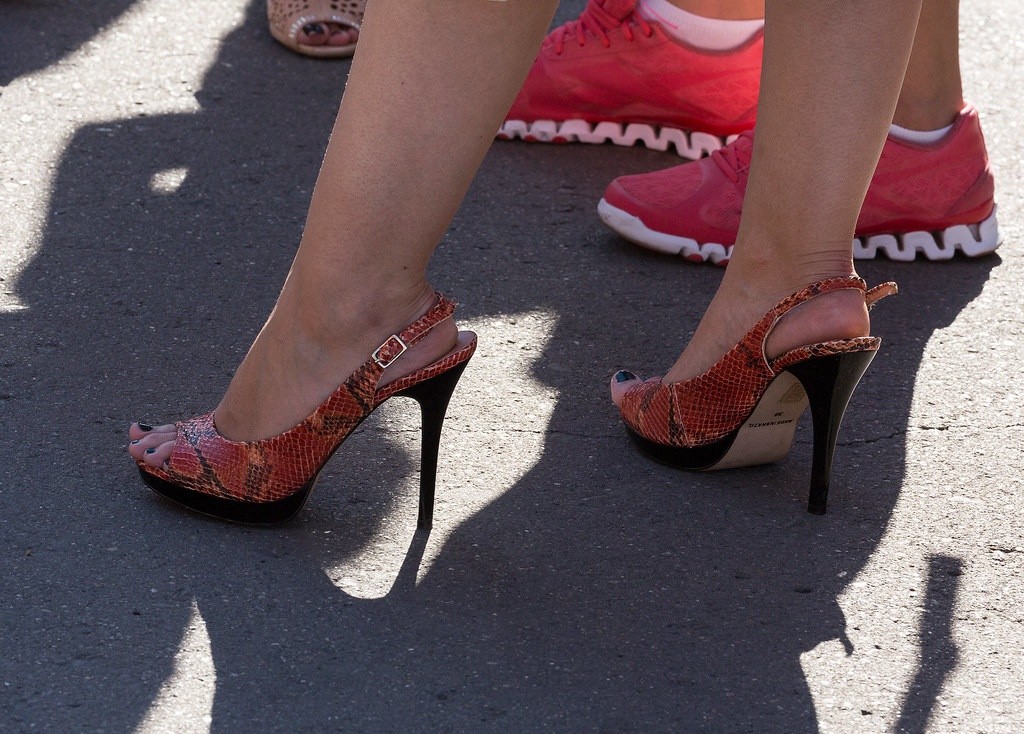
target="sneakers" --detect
[495,0,764,160]
[597,103,1005,262]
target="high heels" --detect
[134,290,478,530]
[617,275,899,515]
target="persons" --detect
[495,0,1003,260]
[124,0,925,530]
[266,0,367,57]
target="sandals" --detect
[265,0,367,58]
